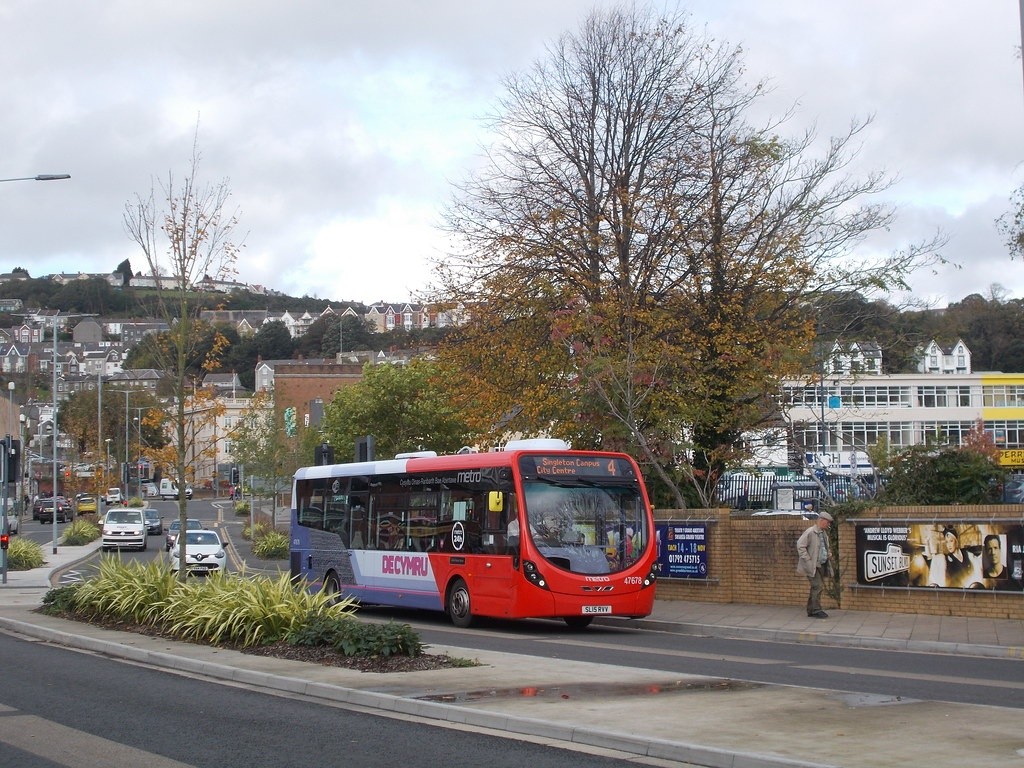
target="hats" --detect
[942,527,959,542]
[819,512,835,521]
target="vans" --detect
[160,478,193,500]
[98,508,150,553]
[0,496,20,536]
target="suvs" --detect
[39,499,74,524]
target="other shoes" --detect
[808,611,828,618]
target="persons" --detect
[24,489,64,515]
[796,512,834,618]
[507,513,543,545]
[928,525,1024,592]
[229,483,241,500]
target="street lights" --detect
[8,381,15,458]
[104,389,146,501]
[119,406,153,502]
[69,369,127,522]
[19,413,25,480]
[22,312,99,555]
[104,438,111,493]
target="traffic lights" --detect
[0,534,9,549]
[231,467,239,485]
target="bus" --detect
[289,438,659,628]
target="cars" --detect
[33,479,160,521]
[715,464,889,509]
[142,508,165,535]
[164,518,209,552]
[166,529,229,578]
[76,496,97,516]
